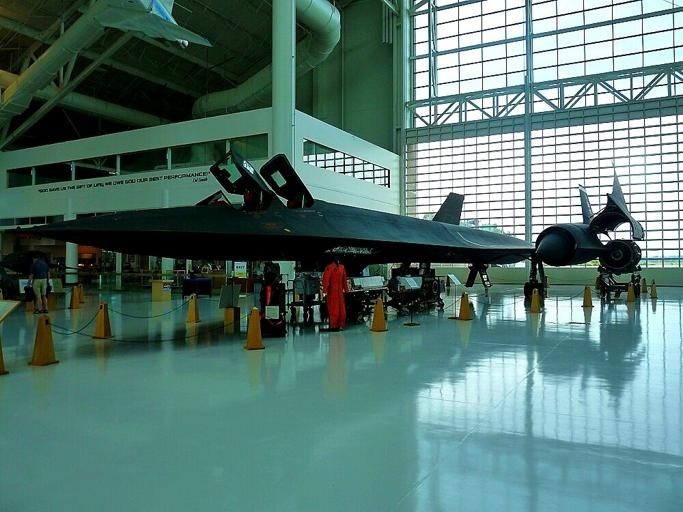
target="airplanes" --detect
[520,163,647,299]
[9,148,537,326]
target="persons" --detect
[321,255,349,330]
[27,257,50,313]
[598,266,615,302]
[318,335,349,403]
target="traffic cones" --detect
[626,281,636,302]
[528,288,542,314]
[0,282,115,375]
[580,286,595,308]
[186,292,201,325]
[241,306,265,351]
[640,277,647,293]
[456,291,473,320]
[369,298,387,332]
[648,278,658,298]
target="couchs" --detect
[182,278,212,299]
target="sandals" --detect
[42,310,48,312]
[33,310,40,314]
